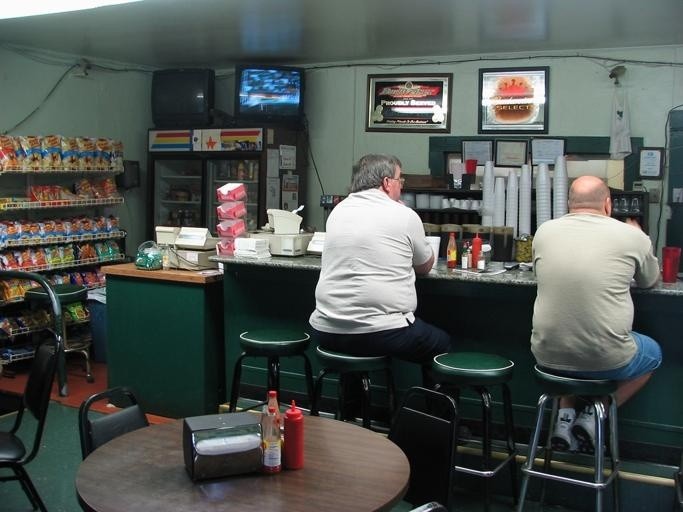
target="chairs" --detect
[409,501,445,512]
[78,384,152,461]
[0,327,63,511]
[382,387,457,512]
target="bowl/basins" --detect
[470,244,491,264]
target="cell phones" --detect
[504,262,519,270]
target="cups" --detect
[553,155,568,219]
[465,160,477,175]
[481,160,532,236]
[612,197,641,213]
[415,195,445,211]
[535,162,552,230]
[661,247,682,283]
[441,198,481,211]
[425,236,442,265]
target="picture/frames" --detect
[476,65,550,135]
[363,71,453,136]
[458,137,566,170]
[635,146,663,181]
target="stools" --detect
[313,342,402,432]
[24,285,96,399]
[433,350,522,509]
[229,327,316,417]
[517,364,622,511]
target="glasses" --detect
[386,176,406,186]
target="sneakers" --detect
[570,402,607,457]
[551,420,579,455]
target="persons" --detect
[307,153,459,420]
[530,175,663,456]
[284,83,297,94]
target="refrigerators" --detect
[142,129,306,244]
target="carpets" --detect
[0,397,111,511]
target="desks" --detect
[74,414,413,512]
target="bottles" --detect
[162,241,171,270]
[461,231,485,270]
[267,390,281,429]
[262,405,284,474]
[247,190,257,230]
[446,233,457,268]
[284,398,305,471]
[218,160,260,181]
[136,241,162,269]
[162,208,199,227]
[166,184,200,202]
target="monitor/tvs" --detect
[151,68,215,129]
[235,64,304,126]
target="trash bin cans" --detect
[86,287,107,363]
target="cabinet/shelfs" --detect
[398,187,651,234]
[1,164,128,364]
